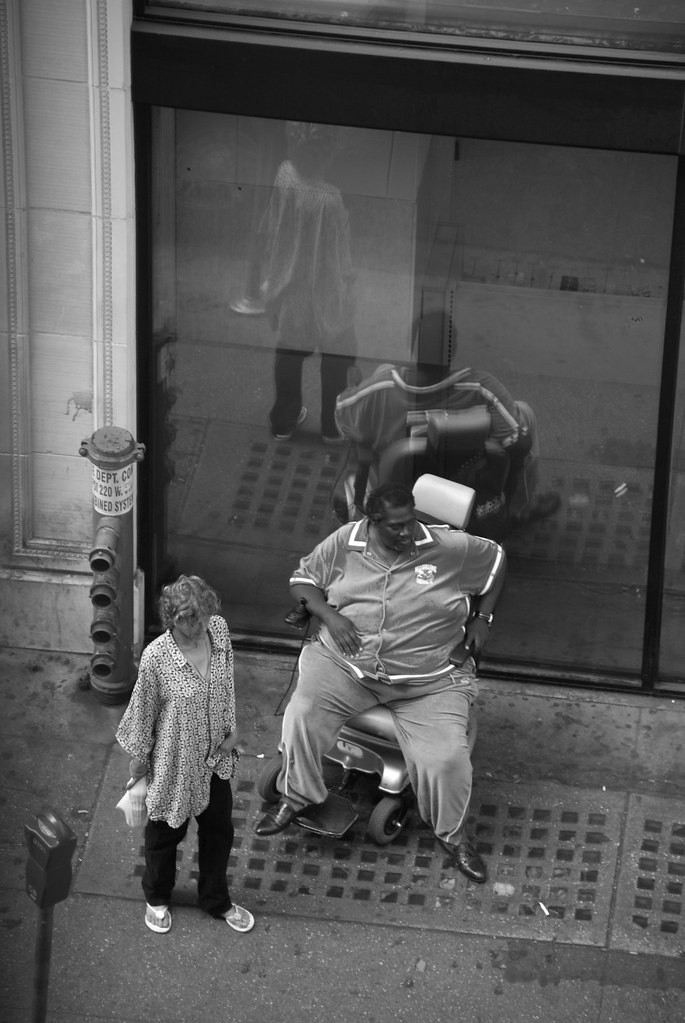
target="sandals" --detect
[144,901,171,933]
[321,433,346,446]
[275,406,308,442]
[221,904,255,932]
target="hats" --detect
[116,775,147,827]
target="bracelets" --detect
[129,759,147,777]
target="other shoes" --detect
[514,492,559,525]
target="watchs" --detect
[472,609,495,627]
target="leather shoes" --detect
[255,799,322,835]
[437,838,486,883]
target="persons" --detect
[114,574,255,934]
[264,120,359,446]
[334,311,560,529]
[255,484,507,884]
